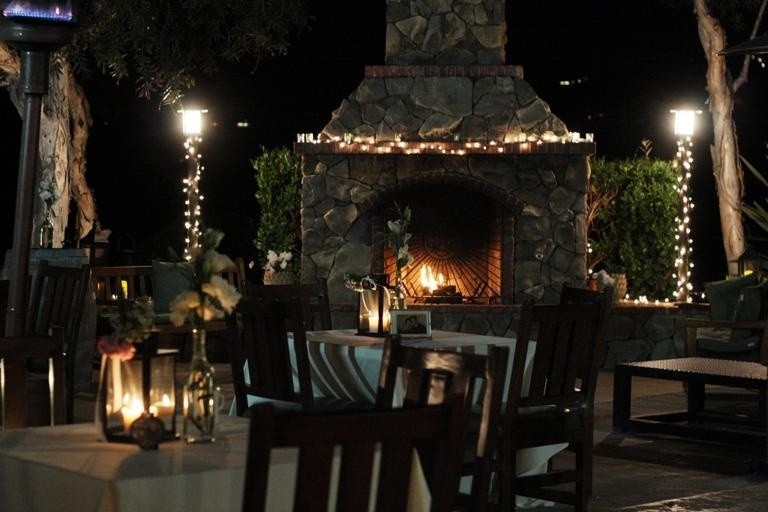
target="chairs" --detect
[0,259,89,430]
[675,272,768,401]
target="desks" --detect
[0,414,414,511]
[613,356,767,451]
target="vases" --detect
[94,354,146,444]
[182,328,219,446]
[40,208,53,248]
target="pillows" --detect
[703,273,768,342]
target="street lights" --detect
[175,94,211,260]
[669,98,705,299]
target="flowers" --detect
[386,199,414,311]
[261,249,293,275]
[152,227,243,436]
[96,315,150,362]
[39,178,59,245]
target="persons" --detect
[402,317,416,330]
[411,316,424,329]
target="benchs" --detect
[92,256,252,369]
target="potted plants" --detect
[608,264,628,299]
